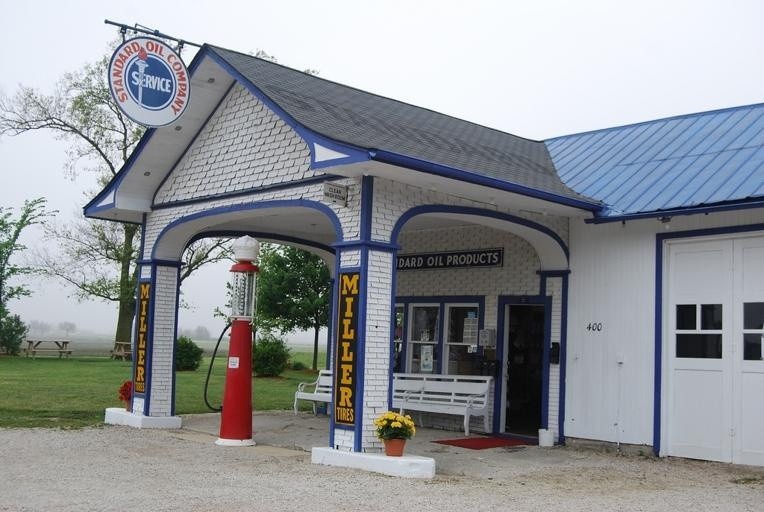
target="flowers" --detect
[374,409,416,438]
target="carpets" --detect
[430,437,538,450]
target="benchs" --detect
[109,349,133,361]
[291,369,491,437]
[23,348,74,360]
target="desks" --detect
[26,339,72,360]
[112,342,133,362]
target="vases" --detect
[382,438,407,455]
